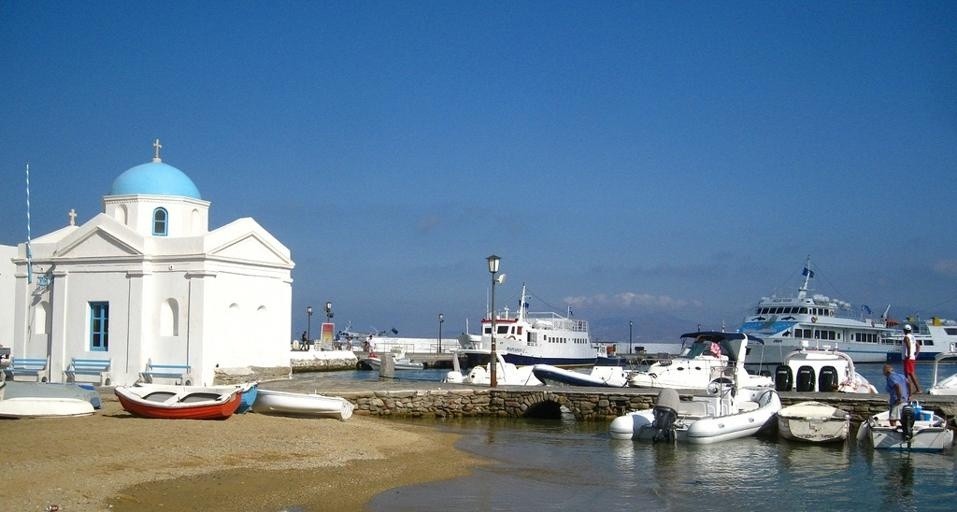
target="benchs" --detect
[4,354,50,384]
[140,358,193,386]
[64,358,113,387]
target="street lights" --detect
[325,301,334,323]
[438,314,444,352]
[306,306,313,349]
[486,254,505,387]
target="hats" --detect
[904,324,912,330]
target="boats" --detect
[356,357,427,370]
[0,397,96,417]
[449,282,626,368]
[115,382,353,421]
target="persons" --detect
[336,331,352,351]
[883,365,912,426]
[368,335,375,353]
[300,331,307,351]
[902,324,923,400]
